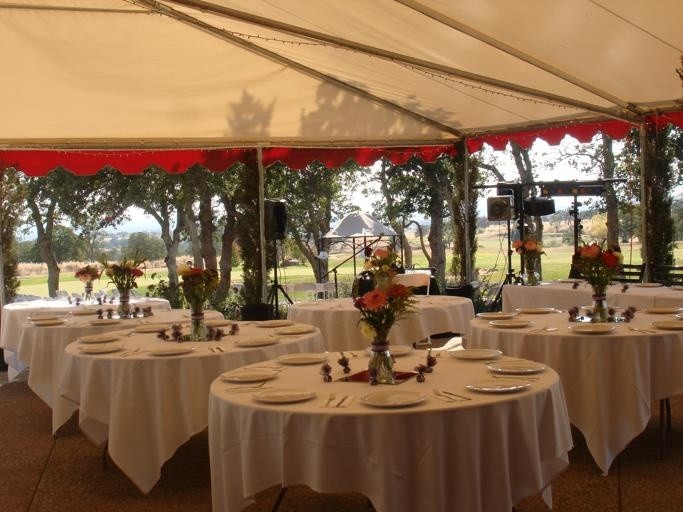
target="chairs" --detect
[393,270,431,295]
[315,284,326,302]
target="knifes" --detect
[527,326,560,332]
[441,385,471,403]
[122,350,150,357]
[216,345,225,353]
[334,394,349,408]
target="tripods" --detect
[490,219,517,312]
[267,241,294,320]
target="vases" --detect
[84,288,95,305]
[593,294,609,322]
[190,314,206,341]
[526,269,542,285]
[368,347,394,385]
[118,297,132,318]
[378,275,390,290]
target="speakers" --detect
[264,198,289,241]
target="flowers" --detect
[514,238,546,283]
[363,238,405,277]
[176,261,215,332]
[99,251,146,314]
[351,283,419,380]
[571,239,630,322]
[75,265,102,299]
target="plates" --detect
[27,314,64,326]
[636,283,661,287]
[568,325,615,333]
[464,380,532,393]
[367,344,412,355]
[135,325,169,332]
[653,321,683,330]
[278,353,326,364]
[516,307,553,313]
[80,336,119,344]
[561,279,582,283]
[255,320,292,327]
[90,319,119,325]
[236,338,279,347]
[485,361,545,374]
[476,312,519,320]
[360,389,429,407]
[222,368,280,383]
[149,346,195,356]
[450,349,503,360]
[275,326,315,334]
[251,388,317,404]
[78,344,122,353]
[488,320,531,328]
[646,307,683,314]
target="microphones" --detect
[378,232,385,239]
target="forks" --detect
[322,390,337,407]
[225,380,266,389]
[119,344,140,355]
[627,325,656,334]
[433,388,457,408]
[533,325,549,336]
[207,345,213,353]
[116,330,134,336]
